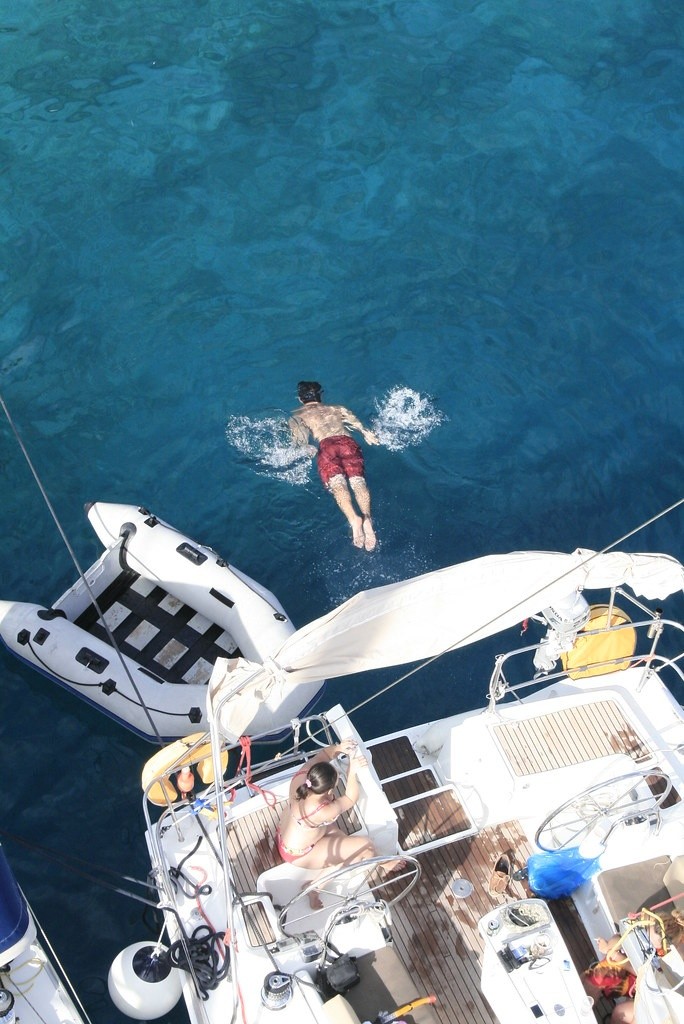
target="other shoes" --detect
[488,854,511,896]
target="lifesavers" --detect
[142,731,232,807]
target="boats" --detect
[0,501,326,748]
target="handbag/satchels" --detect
[527,838,607,899]
[327,954,360,992]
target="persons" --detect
[273,737,407,873]
[581,906,684,1010]
[284,379,383,553]
[603,999,634,1024]
